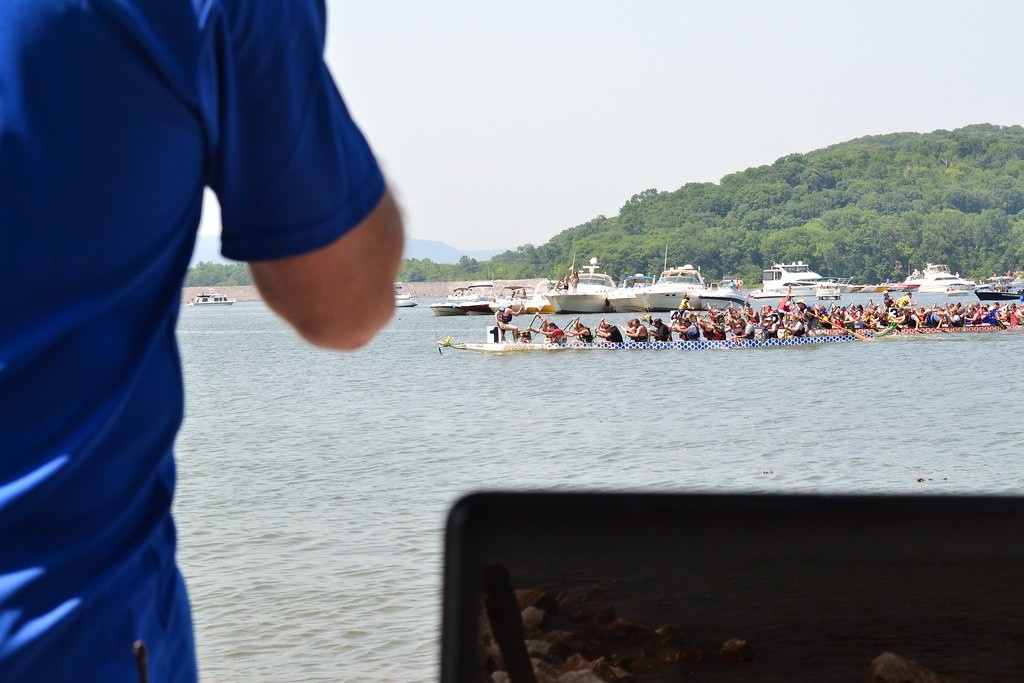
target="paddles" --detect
[851,308,860,318]
[529,315,537,328]
[699,303,716,322]
[807,312,867,340]
[718,302,731,324]
[864,300,871,310]
[566,316,580,334]
[697,305,707,316]
[845,301,853,310]
[563,318,574,331]
[624,334,627,343]
[647,315,651,342]
[669,314,680,333]
[962,306,967,331]
[756,324,770,338]
[593,317,606,342]
[827,302,833,311]
[531,320,545,343]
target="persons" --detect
[566,317,594,343]
[632,279,637,287]
[521,288,526,299]
[554,271,579,293]
[511,291,516,301]
[618,319,650,342]
[672,291,1024,343]
[496,304,523,342]
[596,321,623,342]
[0,0,406,683]
[647,317,670,341]
[728,279,740,289]
[528,312,564,344]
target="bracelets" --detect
[539,316,541,318]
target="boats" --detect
[430,281,561,316]
[635,243,707,311]
[193,289,236,306]
[753,324,1024,337]
[542,250,616,314]
[945,282,970,297]
[699,278,751,311]
[918,263,976,294]
[393,281,418,308]
[815,277,842,301]
[749,247,851,298]
[974,276,1024,301]
[436,332,877,352]
[607,273,656,314]
[847,269,924,293]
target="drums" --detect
[518,331,531,344]
